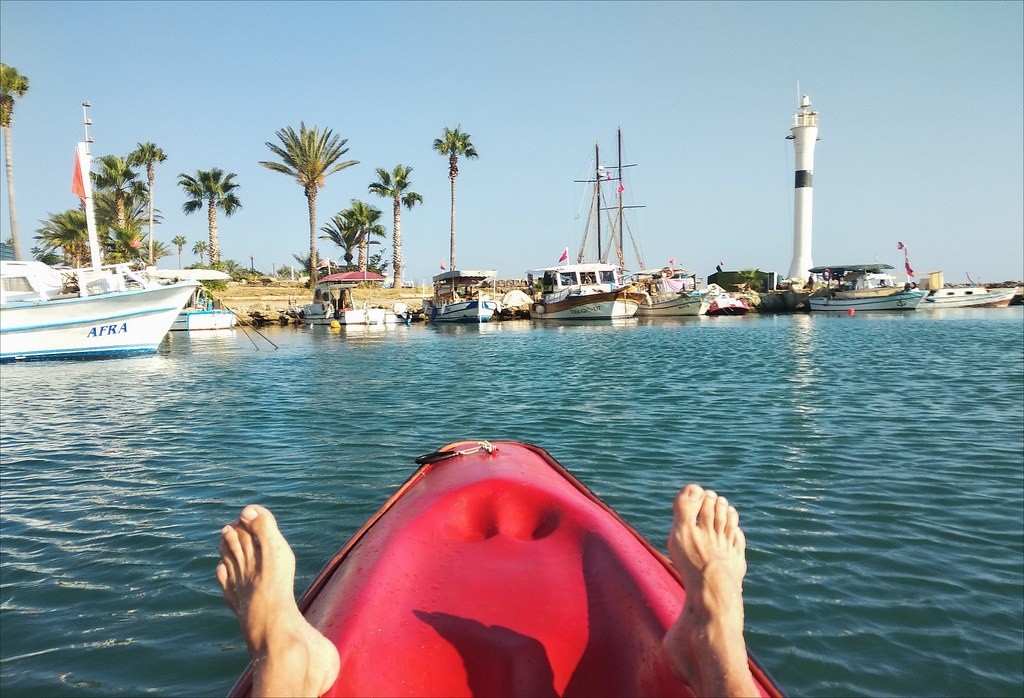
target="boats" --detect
[808,263,930,311]
[168,289,237,331]
[705,283,749,316]
[919,272,1018,310]
[524,261,647,320]
[424,270,500,323]
[0,100,201,361]
[301,271,406,323]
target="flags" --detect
[898,242,914,277]
[559,250,567,262]
[71,148,86,205]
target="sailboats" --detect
[578,126,712,317]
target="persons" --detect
[216,483,761,698]
